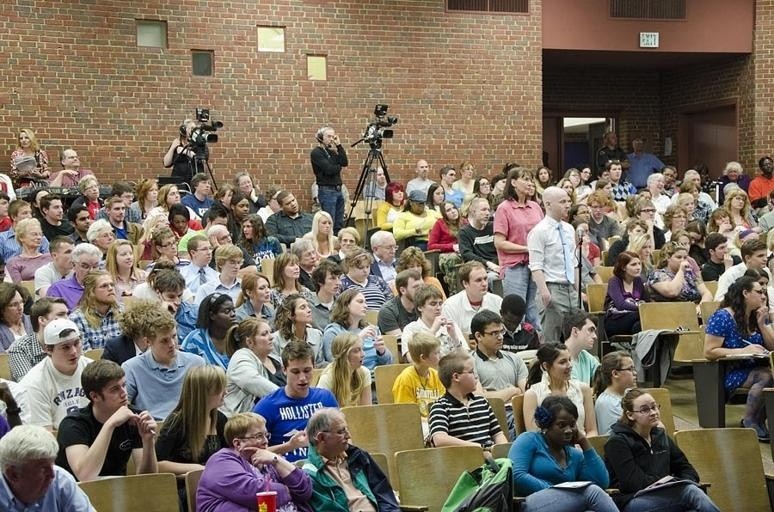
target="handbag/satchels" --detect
[609,298,646,314]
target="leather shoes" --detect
[740,419,771,444]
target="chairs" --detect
[0,198,774,511]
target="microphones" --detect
[316,137,332,149]
[180,131,186,138]
[576,226,585,241]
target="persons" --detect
[508,395,619,512]
[155,364,228,511]
[0,128,774,482]
[0,424,96,512]
[605,388,719,512]
[196,412,312,512]
[163,120,209,189]
[303,408,400,511]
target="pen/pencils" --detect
[742,339,752,345]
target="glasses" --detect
[638,207,657,213]
[625,404,660,415]
[161,233,243,265]
[83,185,100,191]
[478,329,507,338]
[612,366,639,374]
[7,300,26,306]
[236,432,272,442]
[322,426,347,434]
[380,244,399,252]
[463,369,475,375]
[672,214,687,219]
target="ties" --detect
[558,224,575,284]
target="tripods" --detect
[346,149,392,249]
[187,160,217,194]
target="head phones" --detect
[317,132,323,142]
[178,125,186,134]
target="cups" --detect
[254,491,278,512]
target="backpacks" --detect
[441,457,514,512]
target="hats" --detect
[43,318,81,346]
[410,190,427,202]
